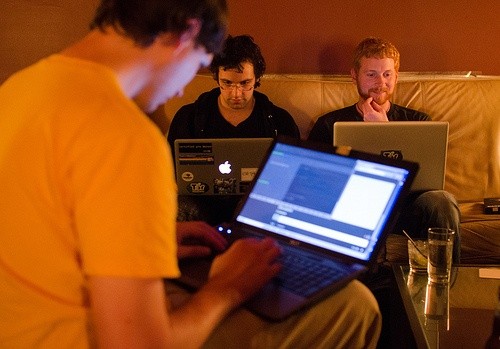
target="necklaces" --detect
[357,102,390,114]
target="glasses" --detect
[217,78,258,90]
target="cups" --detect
[424,279,449,332]
[408,270,428,304]
[428,228,455,279]
[408,240,428,270]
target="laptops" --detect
[333,121,449,192]
[170,136,420,324]
[174,138,275,196]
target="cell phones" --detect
[484,198,500,214]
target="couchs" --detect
[134,75,498,268]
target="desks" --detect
[393,262,500,349]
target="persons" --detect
[0,0,381,349]
[167,33,302,222]
[304,36,461,263]
